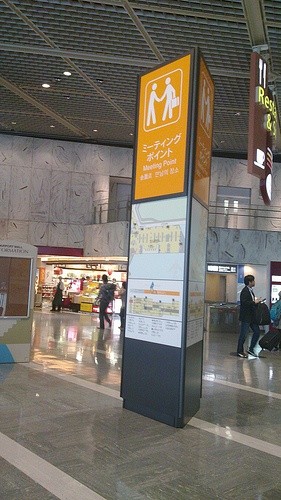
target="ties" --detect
[251,290,255,301]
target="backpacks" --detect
[103,287,114,301]
[59,281,64,291]
[270,299,281,320]
[256,300,271,325]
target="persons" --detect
[270,291,281,352]
[237,275,260,357]
[116,281,129,330]
[97,273,115,330]
[82,276,92,282]
[49,276,65,311]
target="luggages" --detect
[258,330,281,351]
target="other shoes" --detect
[246,347,257,357]
[109,319,111,327]
[57,308,61,311]
[97,326,104,329]
[50,309,56,311]
[237,352,247,358]
